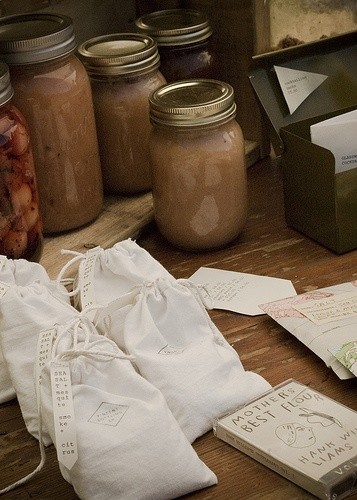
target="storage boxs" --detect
[245,30,357,256]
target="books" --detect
[213,377,357,497]
